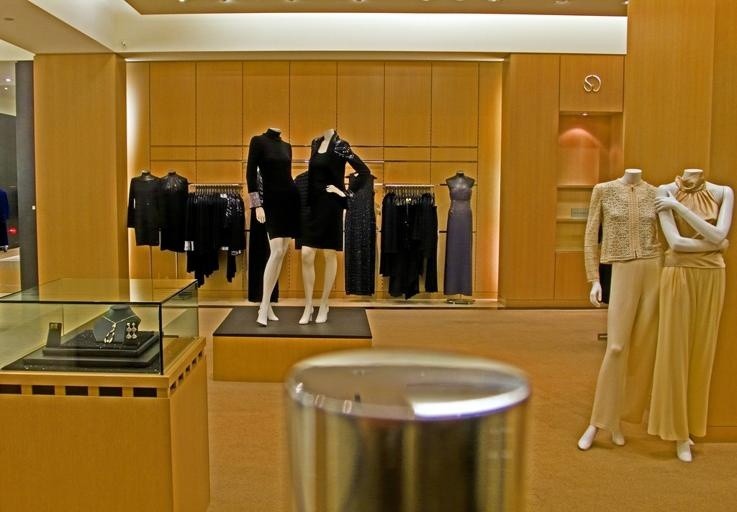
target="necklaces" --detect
[101,314,136,344]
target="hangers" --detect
[192,184,241,205]
[384,184,434,204]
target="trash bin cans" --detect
[286,348,532,512]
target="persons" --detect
[126,170,160,247]
[442,169,479,300]
[647,167,735,466]
[158,169,189,253]
[245,126,298,328]
[576,165,665,453]
[298,126,373,326]
